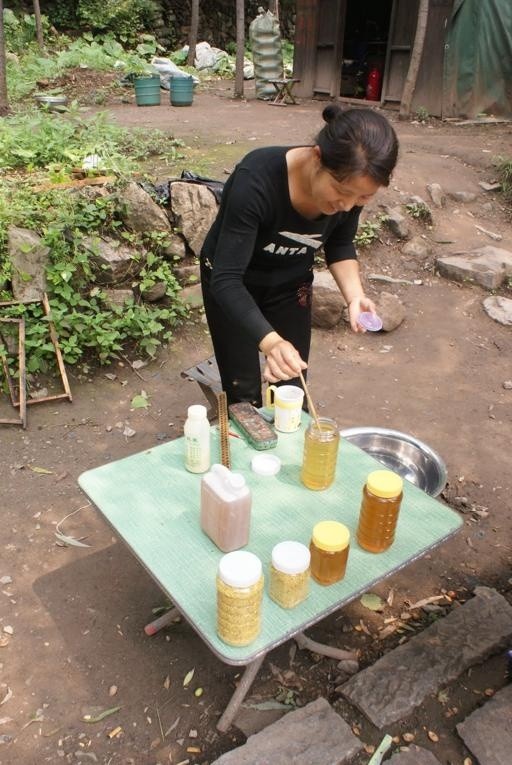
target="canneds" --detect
[355,469,405,554]
[309,521,351,587]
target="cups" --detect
[265,384,304,434]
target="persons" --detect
[200,104,398,418]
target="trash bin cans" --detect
[135,78,160,105]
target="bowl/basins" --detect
[35,97,68,113]
[338,427,447,500]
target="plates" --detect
[251,453,282,477]
[358,311,384,332]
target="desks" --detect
[77,399,464,733]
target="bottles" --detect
[366,67,380,101]
[300,417,339,492]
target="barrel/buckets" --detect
[200,464,251,553]
[134,76,161,106]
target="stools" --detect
[267,78,302,107]
[179,355,263,421]
